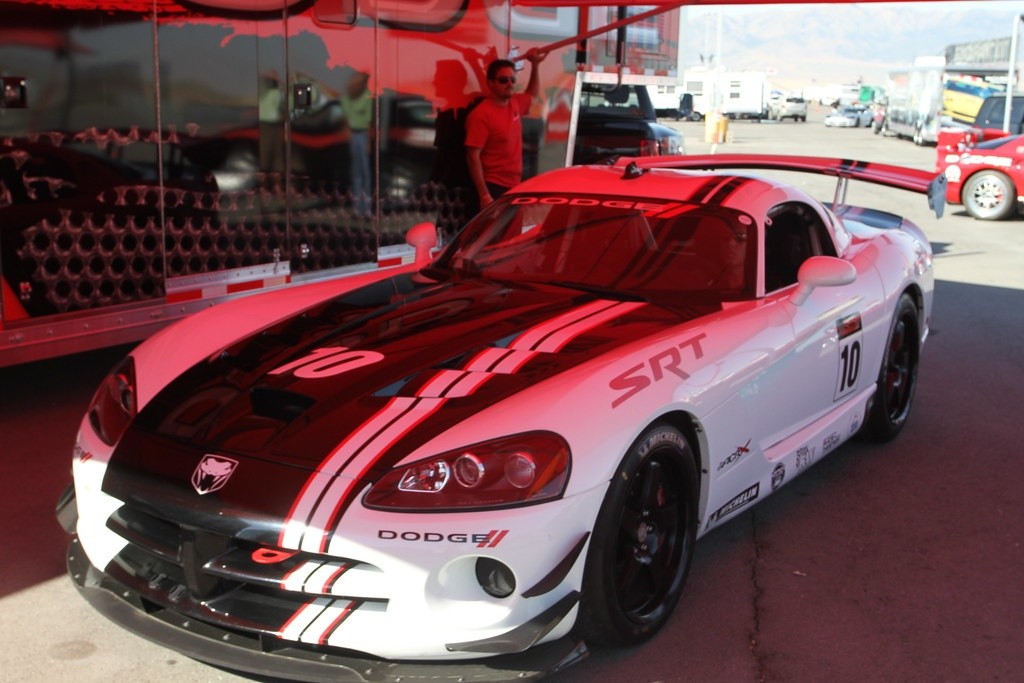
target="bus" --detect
[884,65,944,147]
[944,36,1024,124]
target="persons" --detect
[258,67,282,175]
[468,46,548,208]
[332,72,374,223]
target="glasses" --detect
[492,77,516,83]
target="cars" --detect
[937,94,1024,221]
[56,152,946,683]
[825,106,875,128]
[768,95,807,122]
